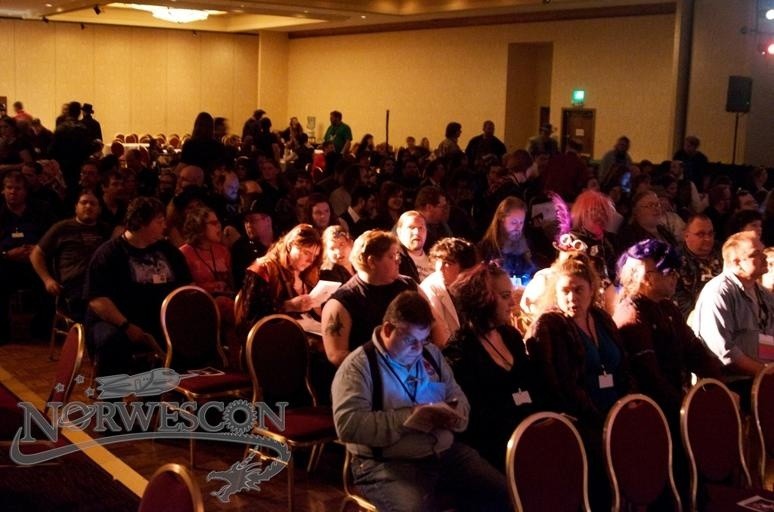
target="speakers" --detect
[725,75,752,114]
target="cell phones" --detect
[446,397,459,408]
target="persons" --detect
[328,289,518,512]
[611,239,742,510]
[1,97,773,414]
[432,261,579,475]
[526,251,626,473]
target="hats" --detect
[81,104,94,114]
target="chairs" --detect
[680,378,774,512]
[0,321,85,483]
[139,463,203,512]
[48,255,80,361]
[82,299,167,433]
[602,394,682,512]
[153,286,253,467]
[237,314,335,512]
[339,448,379,512]
[751,362,774,497]
[506,412,592,512]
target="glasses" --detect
[559,233,588,252]
